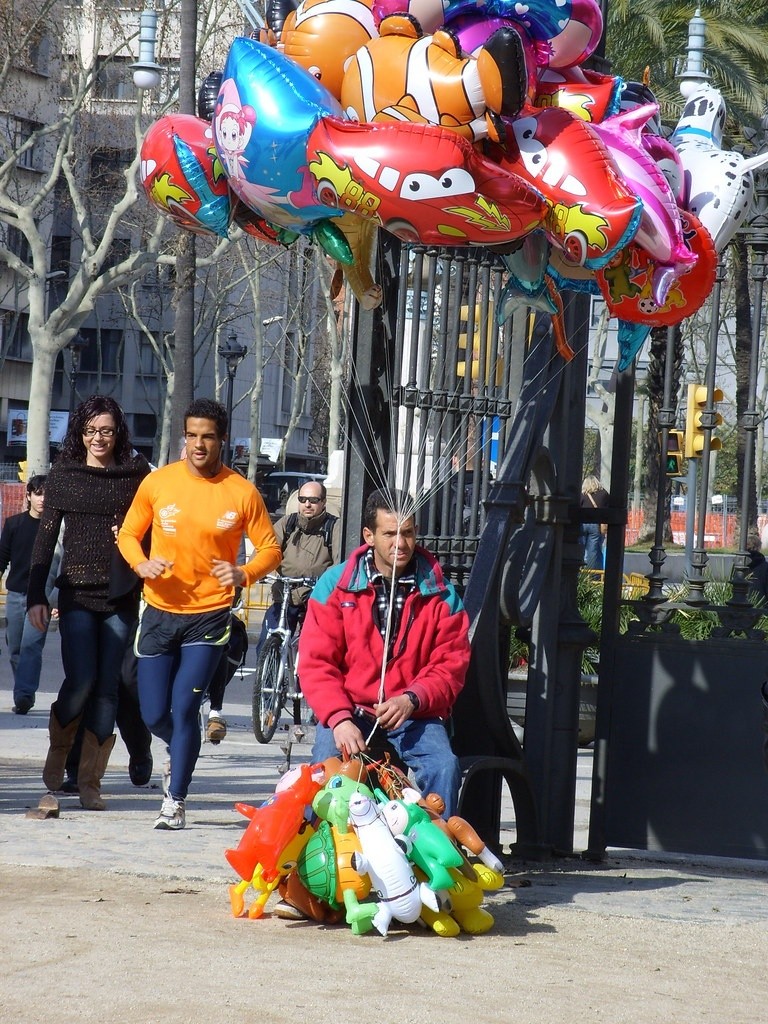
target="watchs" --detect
[403,691,419,711]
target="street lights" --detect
[727,119,768,619]
[219,330,249,470]
[63,332,90,424]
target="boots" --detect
[42,700,86,791]
[78,726,116,810]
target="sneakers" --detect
[155,793,186,830]
[161,747,170,792]
[206,717,226,744]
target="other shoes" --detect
[11,695,34,715]
[61,773,80,792]
[128,751,152,786]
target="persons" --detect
[24,394,283,830]
[579,475,610,581]
[0,474,55,715]
[297,490,469,820]
[247,481,341,709]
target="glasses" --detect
[297,496,321,504]
[81,428,117,436]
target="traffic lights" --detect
[658,430,685,478]
[18,461,28,485]
[457,302,502,389]
[685,383,724,461]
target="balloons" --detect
[139,1,767,370]
[223,743,505,939]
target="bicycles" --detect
[252,571,312,746]
